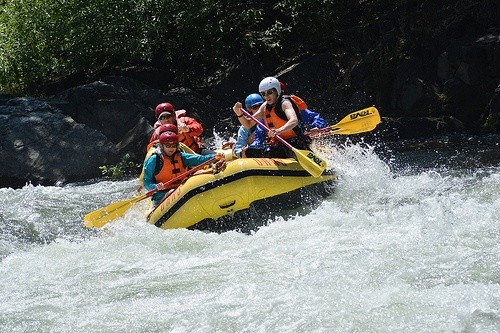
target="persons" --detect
[233,76,306,159]
[139,103,225,207]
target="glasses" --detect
[159,113,173,119]
[163,143,177,147]
[250,104,261,109]
[261,88,276,95]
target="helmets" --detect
[259,76,281,96]
[160,122,178,145]
[155,102,176,119]
[244,93,263,108]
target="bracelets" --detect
[236,113,243,118]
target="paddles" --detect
[305,107,381,136]
[306,125,377,135]
[136,186,143,194]
[84,156,221,228]
[239,107,326,178]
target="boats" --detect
[145,145,343,238]
